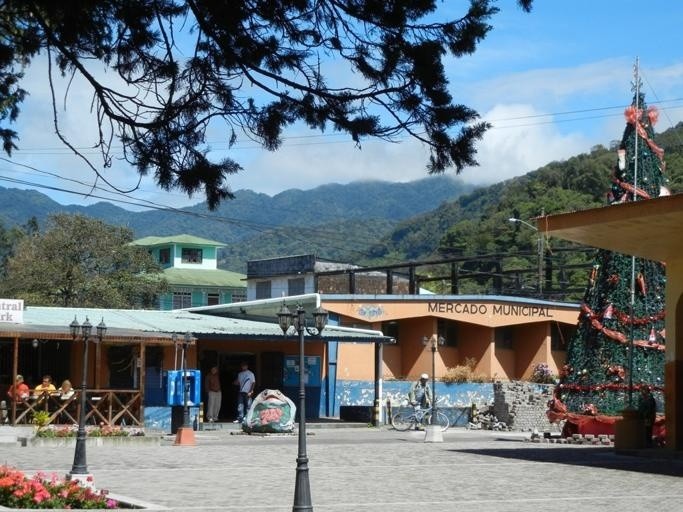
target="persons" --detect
[31,375,60,395]
[5,373,30,405]
[202,364,222,424]
[231,360,255,423]
[637,384,656,448]
[408,373,433,431]
[52,378,75,423]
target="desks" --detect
[20,394,106,426]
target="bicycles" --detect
[391,400,450,432]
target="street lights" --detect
[67,313,107,474]
[171,330,194,428]
[274,295,331,512]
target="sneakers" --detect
[232,419,243,423]
[414,423,426,431]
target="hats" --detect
[421,373,428,380]
